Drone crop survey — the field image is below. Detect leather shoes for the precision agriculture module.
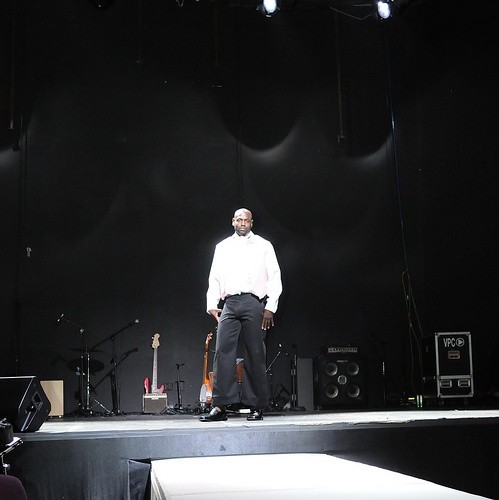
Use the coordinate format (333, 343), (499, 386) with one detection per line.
(247, 408), (263, 421)
(199, 407), (229, 422)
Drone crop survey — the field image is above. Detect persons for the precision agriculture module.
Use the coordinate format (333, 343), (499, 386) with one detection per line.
(200, 207), (282, 422)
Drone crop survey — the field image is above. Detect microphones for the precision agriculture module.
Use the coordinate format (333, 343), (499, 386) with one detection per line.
(57, 314), (64, 322)
(279, 343), (288, 355)
(128, 319), (139, 326)
(80, 328), (85, 337)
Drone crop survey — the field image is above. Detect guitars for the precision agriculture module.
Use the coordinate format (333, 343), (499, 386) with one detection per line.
(143, 332), (165, 393)
(200, 332), (212, 406)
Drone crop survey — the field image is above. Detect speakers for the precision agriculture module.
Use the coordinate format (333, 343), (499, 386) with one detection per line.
(143, 393), (167, 414)
(0, 376), (51, 432)
(317, 353), (368, 410)
